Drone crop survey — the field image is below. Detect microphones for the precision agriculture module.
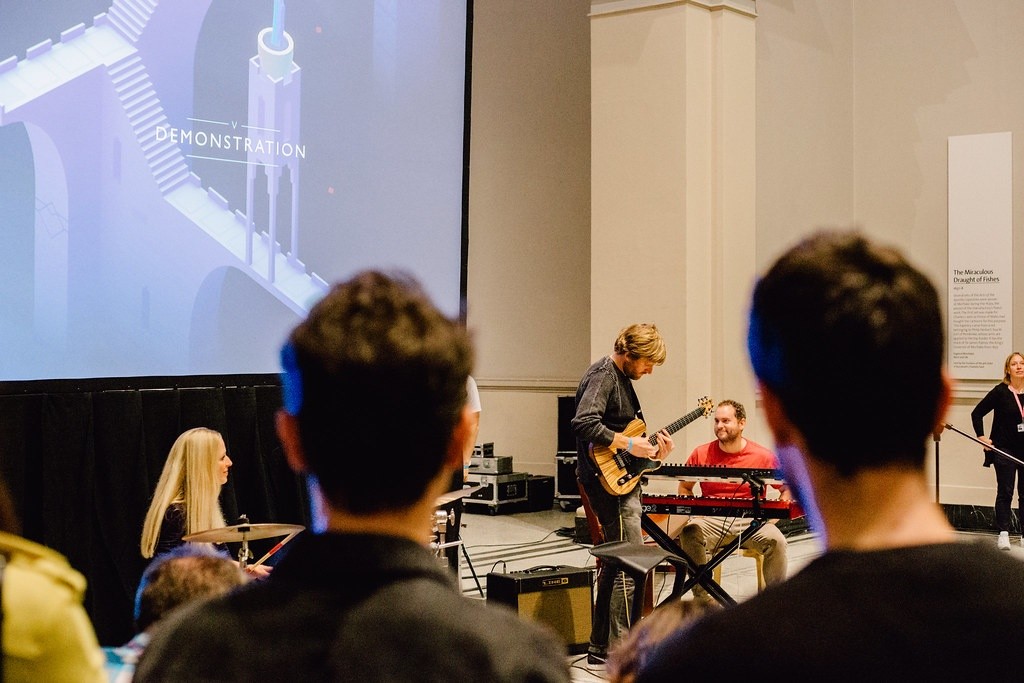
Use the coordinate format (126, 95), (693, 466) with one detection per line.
(742, 472), (761, 488)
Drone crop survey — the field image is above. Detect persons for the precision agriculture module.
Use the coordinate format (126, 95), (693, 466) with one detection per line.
(606, 599), (719, 683)
(571, 324), (676, 671)
(435, 374), (482, 591)
(0, 482), (107, 683)
(131, 271), (573, 683)
(970, 352), (1024, 550)
(138, 427), (274, 581)
(677, 400), (792, 607)
(101, 544), (241, 683)
(633, 231), (1024, 683)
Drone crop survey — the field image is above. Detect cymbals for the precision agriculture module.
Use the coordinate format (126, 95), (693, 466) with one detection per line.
(181, 514), (306, 544)
(433, 485), (487, 507)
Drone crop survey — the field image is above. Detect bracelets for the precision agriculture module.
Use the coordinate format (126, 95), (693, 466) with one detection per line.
(626, 438), (633, 452)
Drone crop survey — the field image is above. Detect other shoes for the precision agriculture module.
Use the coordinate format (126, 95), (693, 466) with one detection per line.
(588, 652), (609, 664)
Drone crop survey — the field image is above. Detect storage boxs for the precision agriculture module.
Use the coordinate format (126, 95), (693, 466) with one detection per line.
(463, 454), (580, 516)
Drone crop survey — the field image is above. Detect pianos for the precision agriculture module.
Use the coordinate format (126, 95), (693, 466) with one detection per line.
(632, 491), (808, 521)
(643, 457), (792, 488)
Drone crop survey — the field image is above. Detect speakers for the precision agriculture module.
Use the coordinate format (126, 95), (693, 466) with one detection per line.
(555, 394), (583, 454)
(486, 565), (596, 657)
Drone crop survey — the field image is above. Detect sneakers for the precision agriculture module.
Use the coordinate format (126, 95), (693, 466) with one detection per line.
(1021, 535), (1024, 547)
(997, 530), (1010, 550)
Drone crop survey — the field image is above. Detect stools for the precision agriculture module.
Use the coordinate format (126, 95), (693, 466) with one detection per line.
(711, 547), (765, 597)
(588, 540), (689, 665)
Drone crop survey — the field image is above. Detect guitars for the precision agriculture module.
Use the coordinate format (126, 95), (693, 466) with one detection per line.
(588, 395), (716, 496)
